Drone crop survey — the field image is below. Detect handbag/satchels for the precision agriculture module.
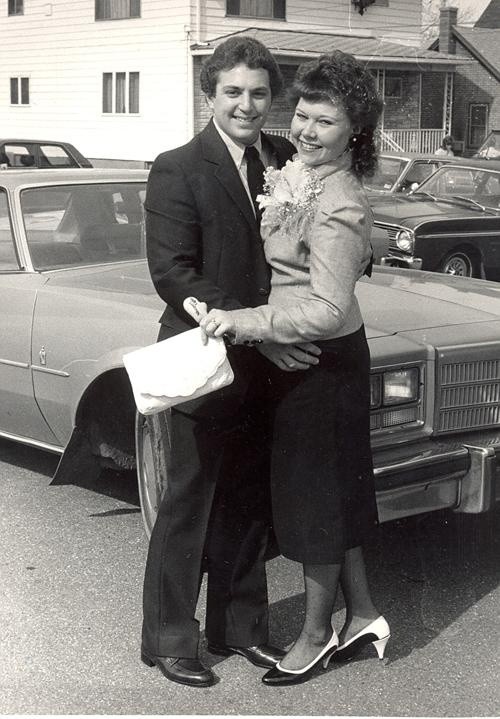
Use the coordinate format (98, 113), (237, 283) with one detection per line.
(335, 615), (391, 661)
(122, 324), (236, 417)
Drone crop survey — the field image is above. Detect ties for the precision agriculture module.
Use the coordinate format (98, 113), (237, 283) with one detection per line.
(244, 145), (266, 227)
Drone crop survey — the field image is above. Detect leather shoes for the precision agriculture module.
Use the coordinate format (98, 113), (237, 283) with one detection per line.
(140, 641), (214, 687)
(207, 638), (288, 669)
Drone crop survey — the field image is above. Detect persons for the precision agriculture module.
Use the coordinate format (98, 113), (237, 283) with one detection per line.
(138, 33), (379, 690)
(199, 46), (392, 687)
(433, 134), (457, 173)
(478, 136), (499, 158)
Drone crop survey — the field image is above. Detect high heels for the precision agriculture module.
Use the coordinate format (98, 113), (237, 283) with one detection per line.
(262, 623), (340, 686)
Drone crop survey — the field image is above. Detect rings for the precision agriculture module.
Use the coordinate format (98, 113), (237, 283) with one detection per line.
(289, 362), (296, 368)
(212, 319), (220, 327)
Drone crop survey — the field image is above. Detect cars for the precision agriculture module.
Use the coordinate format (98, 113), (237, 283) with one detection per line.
(376, 151), (482, 195)
(367, 163), (500, 278)
(0, 138), (132, 250)
(0, 167), (499, 547)
